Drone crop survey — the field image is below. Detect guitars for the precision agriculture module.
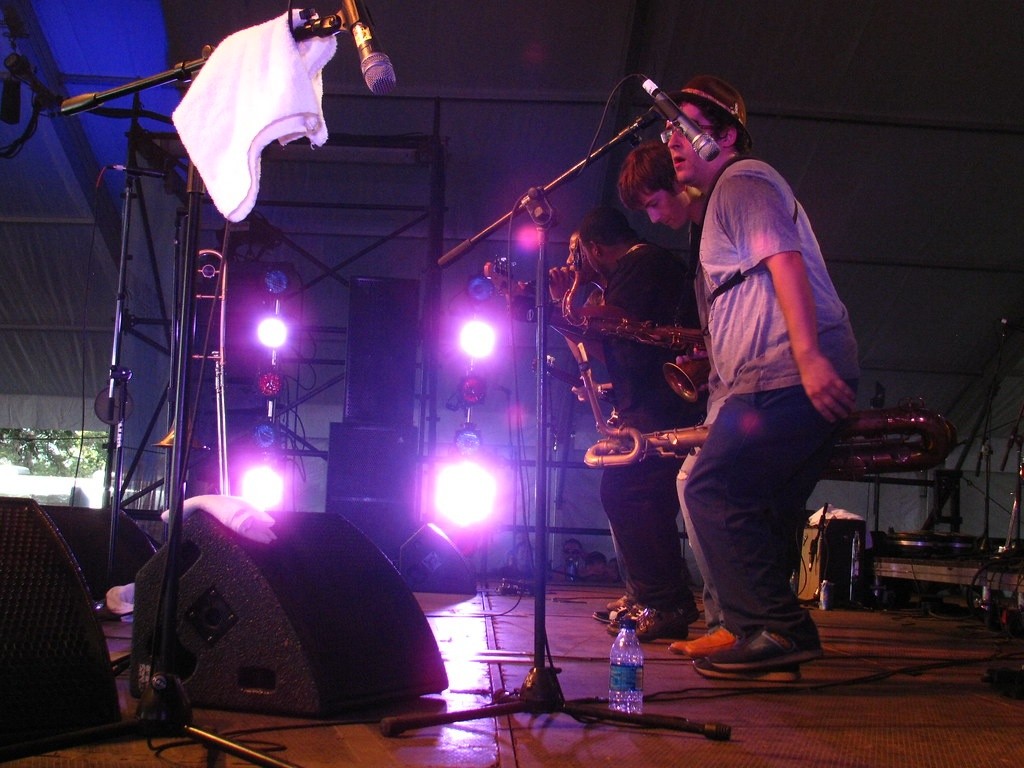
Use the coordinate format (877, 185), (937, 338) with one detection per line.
(483, 253), (608, 367)
(530, 353), (623, 427)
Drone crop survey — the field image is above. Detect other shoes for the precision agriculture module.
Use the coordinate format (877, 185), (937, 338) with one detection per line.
(607, 596), (629, 611)
(685, 627), (739, 659)
(668, 641), (693, 655)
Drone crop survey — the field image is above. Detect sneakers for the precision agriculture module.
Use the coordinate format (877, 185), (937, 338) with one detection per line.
(693, 640), (801, 682)
(607, 605), (700, 642)
(710, 628), (824, 670)
(592, 603), (646, 623)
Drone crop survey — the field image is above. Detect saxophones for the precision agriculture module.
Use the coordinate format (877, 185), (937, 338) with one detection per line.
(561, 236), (711, 403)
(575, 341), (953, 479)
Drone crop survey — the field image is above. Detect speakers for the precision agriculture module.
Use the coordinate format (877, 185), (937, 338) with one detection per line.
(327, 422), (421, 571)
(343, 277), (421, 424)
(0, 497), (478, 765)
(794, 516), (867, 605)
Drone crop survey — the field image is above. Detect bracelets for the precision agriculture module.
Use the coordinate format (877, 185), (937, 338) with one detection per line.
(598, 384), (602, 393)
(553, 296), (564, 304)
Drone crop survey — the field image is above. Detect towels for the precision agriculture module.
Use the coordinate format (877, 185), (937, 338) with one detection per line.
(808, 503), (867, 526)
(158, 493), (279, 546)
(169, 6), (340, 225)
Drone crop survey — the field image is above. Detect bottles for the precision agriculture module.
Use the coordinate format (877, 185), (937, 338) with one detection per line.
(607, 619), (644, 714)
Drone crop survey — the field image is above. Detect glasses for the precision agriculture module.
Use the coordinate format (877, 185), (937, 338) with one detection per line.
(660, 118), (726, 144)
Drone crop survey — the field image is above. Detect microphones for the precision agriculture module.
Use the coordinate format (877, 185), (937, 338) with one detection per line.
(340, 0), (396, 96)
(106, 164), (169, 179)
(809, 542), (816, 571)
(636, 75), (721, 162)
(1000, 316), (1024, 332)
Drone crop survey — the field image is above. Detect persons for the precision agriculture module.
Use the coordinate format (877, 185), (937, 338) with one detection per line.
(497, 75), (863, 682)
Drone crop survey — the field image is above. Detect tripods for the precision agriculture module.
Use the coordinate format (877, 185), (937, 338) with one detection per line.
(0, 0), (373, 768)
(381, 98), (732, 741)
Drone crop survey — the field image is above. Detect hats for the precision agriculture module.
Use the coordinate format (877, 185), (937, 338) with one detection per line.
(667, 74), (753, 155)
